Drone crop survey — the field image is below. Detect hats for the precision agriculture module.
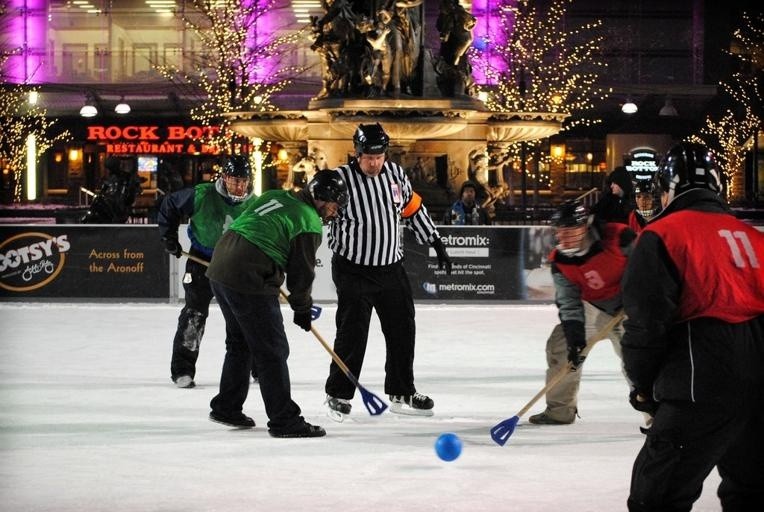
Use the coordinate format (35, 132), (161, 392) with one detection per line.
(459, 181), (477, 191)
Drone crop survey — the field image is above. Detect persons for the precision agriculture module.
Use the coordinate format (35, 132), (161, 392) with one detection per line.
(325, 124), (452, 410)
(203, 168), (350, 439)
(621, 140), (763, 511)
(528, 167), (661, 436)
(442, 181), (492, 225)
(156, 156), (261, 389)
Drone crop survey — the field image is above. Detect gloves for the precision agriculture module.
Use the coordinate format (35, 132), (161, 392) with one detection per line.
(159, 230), (183, 259)
(293, 309), (312, 332)
(568, 336), (587, 369)
(438, 243), (452, 277)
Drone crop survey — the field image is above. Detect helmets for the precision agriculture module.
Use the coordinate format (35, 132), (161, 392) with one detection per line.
(223, 154), (252, 178)
(656, 141), (726, 198)
(352, 121), (391, 155)
(634, 180), (658, 197)
(550, 199), (590, 228)
(308, 169), (349, 209)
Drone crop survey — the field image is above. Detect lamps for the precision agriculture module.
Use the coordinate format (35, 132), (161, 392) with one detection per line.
(79, 89), (98, 119)
(658, 93), (677, 118)
(621, 91), (638, 115)
(113, 90), (130, 115)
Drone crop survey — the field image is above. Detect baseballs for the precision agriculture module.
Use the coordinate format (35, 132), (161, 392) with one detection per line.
(436, 434), (461, 461)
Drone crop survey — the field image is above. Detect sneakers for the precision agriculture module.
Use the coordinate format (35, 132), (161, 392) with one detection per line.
(323, 394), (353, 414)
(529, 412), (571, 424)
(208, 409), (256, 428)
(171, 375), (196, 389)
(388, 392), (434, 409)
(267, 417), (326, 438)
